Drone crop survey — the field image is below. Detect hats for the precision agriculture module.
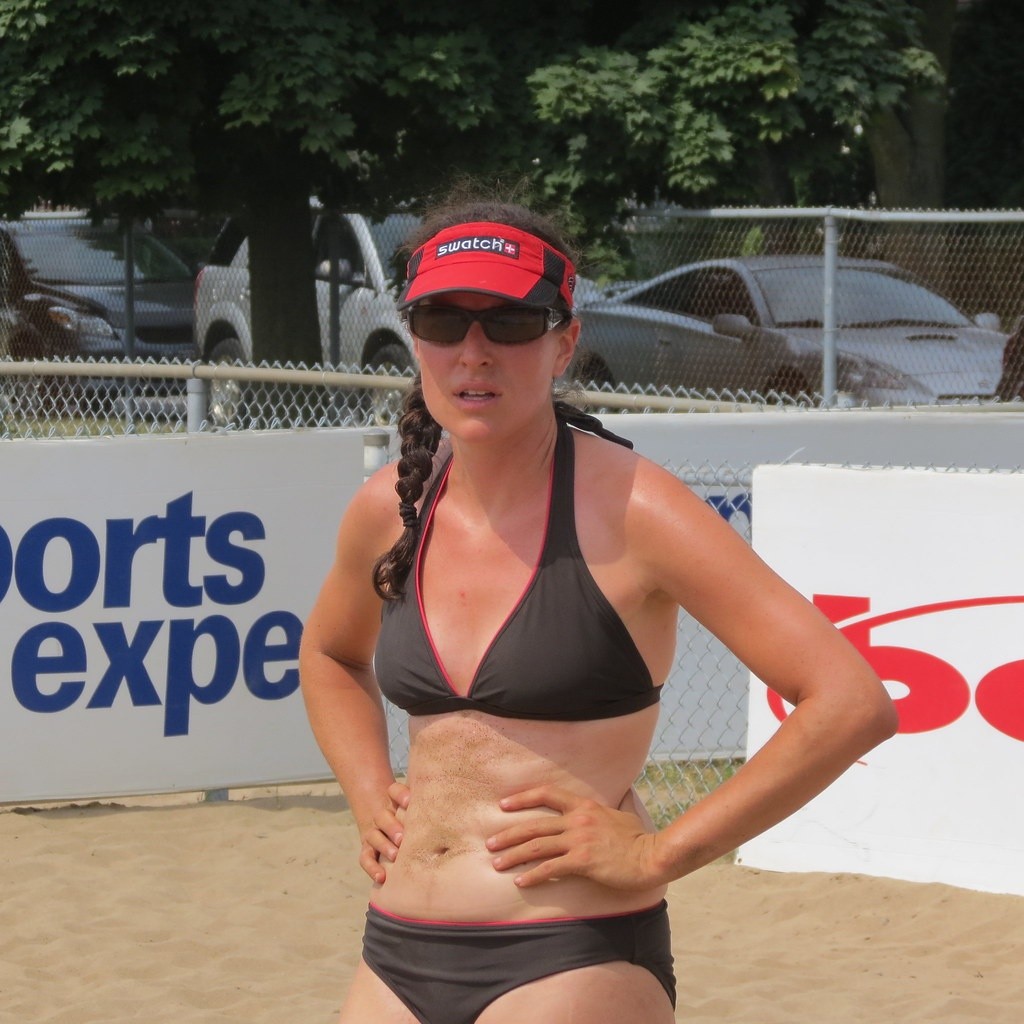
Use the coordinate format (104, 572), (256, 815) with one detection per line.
(397, 221), (578, 311)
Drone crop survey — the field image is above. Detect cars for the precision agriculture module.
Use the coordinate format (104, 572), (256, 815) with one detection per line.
(562, 253), (1012, 408)
(190, 189), (605, 430)
(0, 218), (197, 417)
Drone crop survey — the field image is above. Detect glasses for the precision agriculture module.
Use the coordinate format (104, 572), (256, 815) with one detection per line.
(401, 302), (571, 344)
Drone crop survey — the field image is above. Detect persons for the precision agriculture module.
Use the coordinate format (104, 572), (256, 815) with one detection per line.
(298, 200), (897, 1024)
